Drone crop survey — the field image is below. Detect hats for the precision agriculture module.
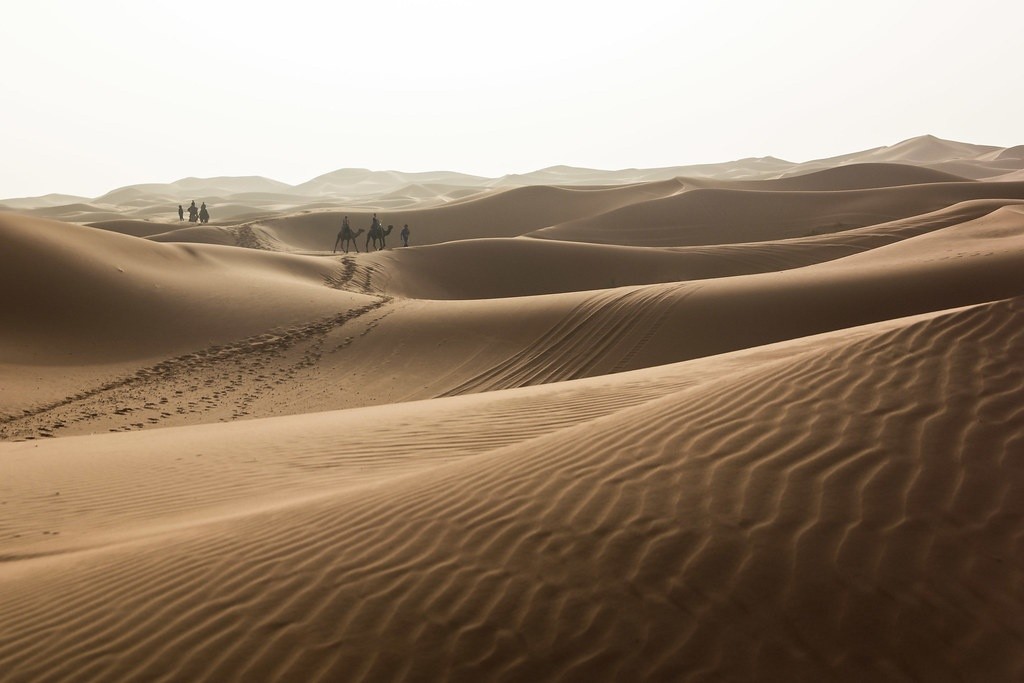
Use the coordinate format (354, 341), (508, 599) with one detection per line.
(404, 225), (408, 227)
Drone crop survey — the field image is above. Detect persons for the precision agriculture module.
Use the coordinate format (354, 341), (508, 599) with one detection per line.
(401, 225), (410, 247)
(372, 213), (380, 235)
(342, 215), (349, 239)
(179, 204), (185, 221)
(191, 200), (196, 207)
(199, 201), (206, 215)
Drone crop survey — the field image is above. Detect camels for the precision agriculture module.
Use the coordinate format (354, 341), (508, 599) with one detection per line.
(333, 228), (365, 254)
(188, 207), (209, 223)
(366, 224), (393, 252)
